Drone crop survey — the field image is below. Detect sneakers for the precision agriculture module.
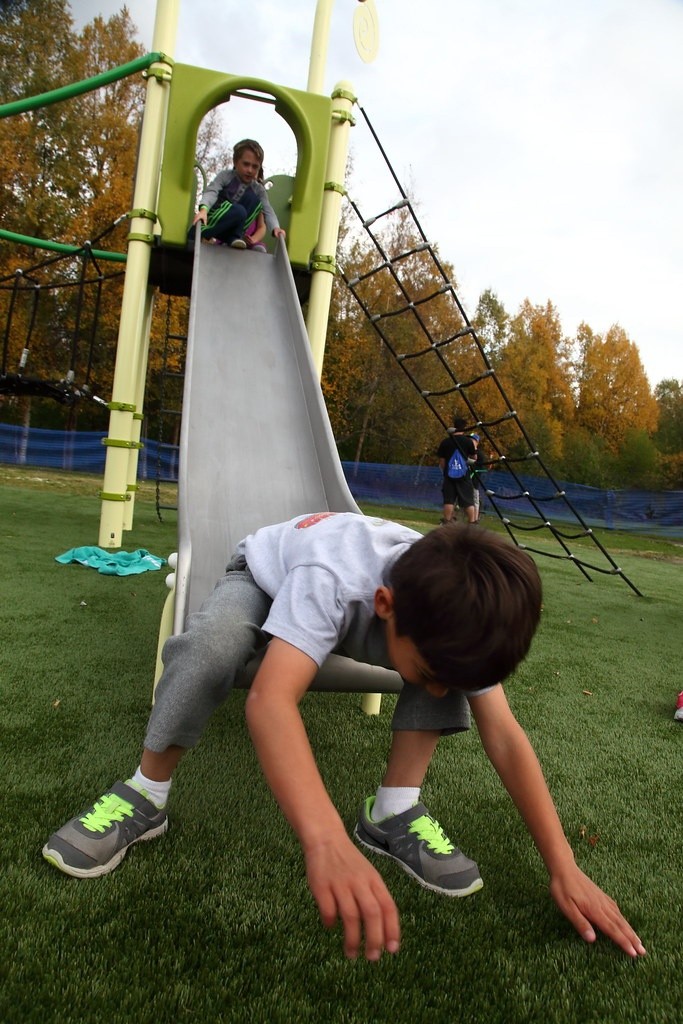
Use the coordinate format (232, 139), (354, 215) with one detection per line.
(353, 796), (483, 898)
(42, 780), (168, 879)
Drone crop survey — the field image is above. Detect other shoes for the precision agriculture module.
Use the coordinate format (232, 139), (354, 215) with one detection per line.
(253, 241), (267, 252)
(467, 519), (479, 528)
(202, 236), (221, 245)
(439, 516), (457, 526)
(231, 239), (246, 249)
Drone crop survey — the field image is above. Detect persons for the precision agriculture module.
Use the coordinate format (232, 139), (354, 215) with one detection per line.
(454, 433), (483, 522)
(437, 418), (480, 528)
(191, 139), (286, 253)
(42, 510), (647, 959)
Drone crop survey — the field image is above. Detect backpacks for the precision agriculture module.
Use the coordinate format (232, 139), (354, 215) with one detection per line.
(444, 437), (469, 479)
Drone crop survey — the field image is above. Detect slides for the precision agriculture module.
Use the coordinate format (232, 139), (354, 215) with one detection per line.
(152, 216), (406, 714)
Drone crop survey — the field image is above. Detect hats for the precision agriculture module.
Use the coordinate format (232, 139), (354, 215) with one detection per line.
(466, 433), (479, 443)
